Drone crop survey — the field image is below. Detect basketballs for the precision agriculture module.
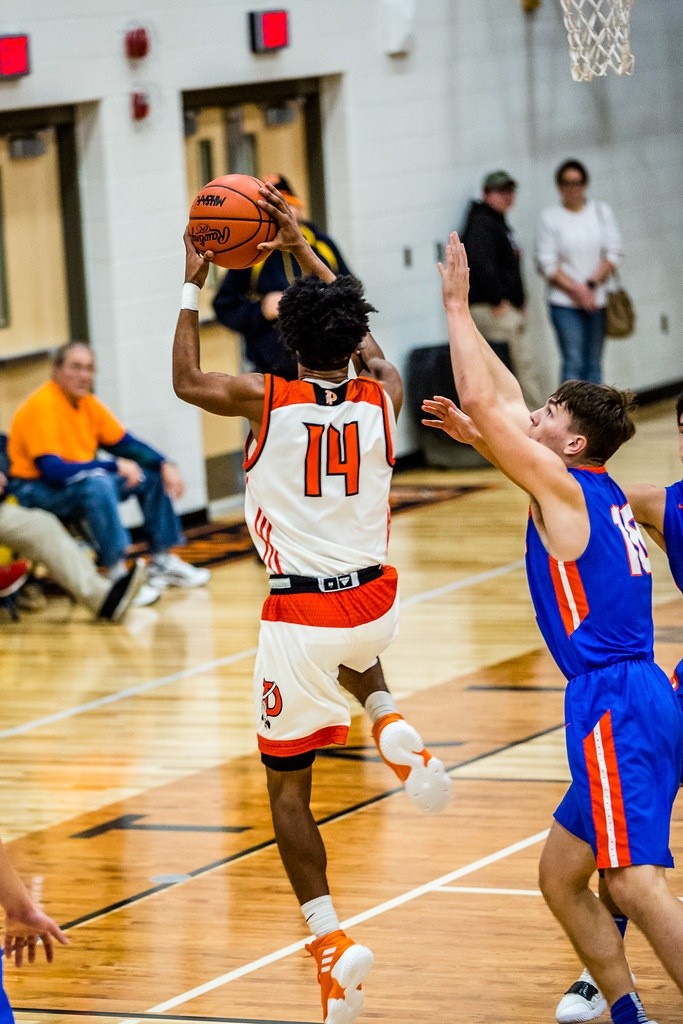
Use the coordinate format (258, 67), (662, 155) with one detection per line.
(188, 173), (278, 271)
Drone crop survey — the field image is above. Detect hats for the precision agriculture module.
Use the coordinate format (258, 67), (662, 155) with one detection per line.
(485, 172), (516, 194)
(262, 174), (304, 210)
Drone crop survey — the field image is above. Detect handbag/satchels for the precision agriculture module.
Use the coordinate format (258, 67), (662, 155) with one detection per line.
(606, 259), (634, 336)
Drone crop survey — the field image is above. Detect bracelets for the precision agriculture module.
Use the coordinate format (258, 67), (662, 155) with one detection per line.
(180, 282), (200, 312)
(585, 277), (598, 292)
(182, 280), (202, 288)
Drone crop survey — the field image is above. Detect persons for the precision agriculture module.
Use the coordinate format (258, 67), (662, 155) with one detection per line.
(420, 379), (683, 1021)
(0, 839), (70, 1023)
(1, 341), (212, 622)
(532, 158), (625, 386)
(171, 181), (451, 1024)
(458, 171), (527, 376)
(211, 175), (371, 383)
(420, 232), (683, 1024)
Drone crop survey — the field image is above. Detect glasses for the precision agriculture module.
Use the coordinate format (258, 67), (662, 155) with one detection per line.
(558, 176), (586, 189)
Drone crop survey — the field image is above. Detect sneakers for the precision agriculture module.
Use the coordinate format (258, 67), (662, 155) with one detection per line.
(147, 553), (211, 589)
(0, 562), (28, 597)
(98, 557), (145, 624)
(556, 957), (636, 1024)
(107, 570), (160, 607)
(305, 928), (374, 1024)
(370, 712), (453, 814)
